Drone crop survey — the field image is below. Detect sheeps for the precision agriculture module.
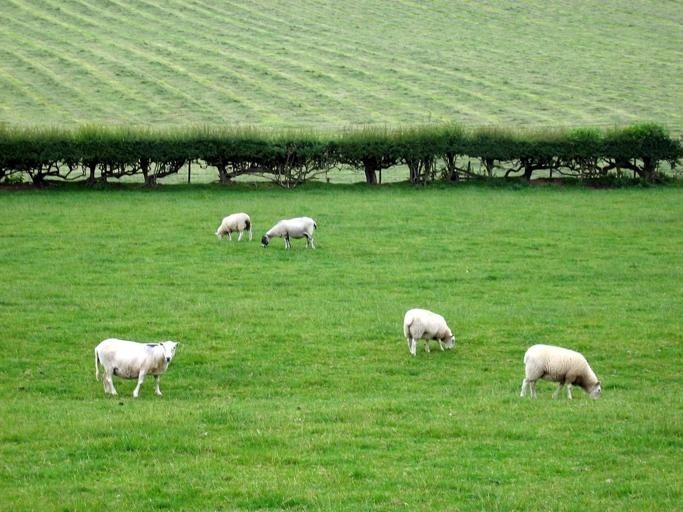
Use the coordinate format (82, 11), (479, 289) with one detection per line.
(261, 216), (317, 250)
(402, 308), (456, 358)
(214, 212), (252, 243)
(519, 344), (600, 401)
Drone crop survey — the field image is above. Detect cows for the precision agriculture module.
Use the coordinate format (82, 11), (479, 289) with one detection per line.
(94, 338), (179, 400)
